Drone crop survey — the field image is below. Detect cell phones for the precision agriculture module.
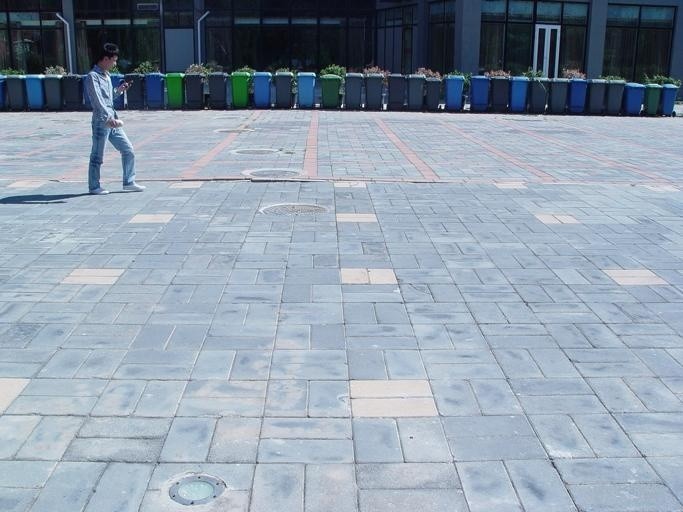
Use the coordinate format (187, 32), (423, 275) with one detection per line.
(125, 80), (133, 88)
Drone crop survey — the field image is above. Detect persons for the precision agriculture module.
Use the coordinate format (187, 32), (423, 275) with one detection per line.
(25, 46), (42, 73)
(83, 42), (146, 195)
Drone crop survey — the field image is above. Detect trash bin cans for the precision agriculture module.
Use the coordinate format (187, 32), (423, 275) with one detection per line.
(0, 71), (680, 117)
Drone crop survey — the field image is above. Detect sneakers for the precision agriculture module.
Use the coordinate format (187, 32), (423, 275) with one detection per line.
(88, 187), (110, 195)
(121, 183), (146, 192)
